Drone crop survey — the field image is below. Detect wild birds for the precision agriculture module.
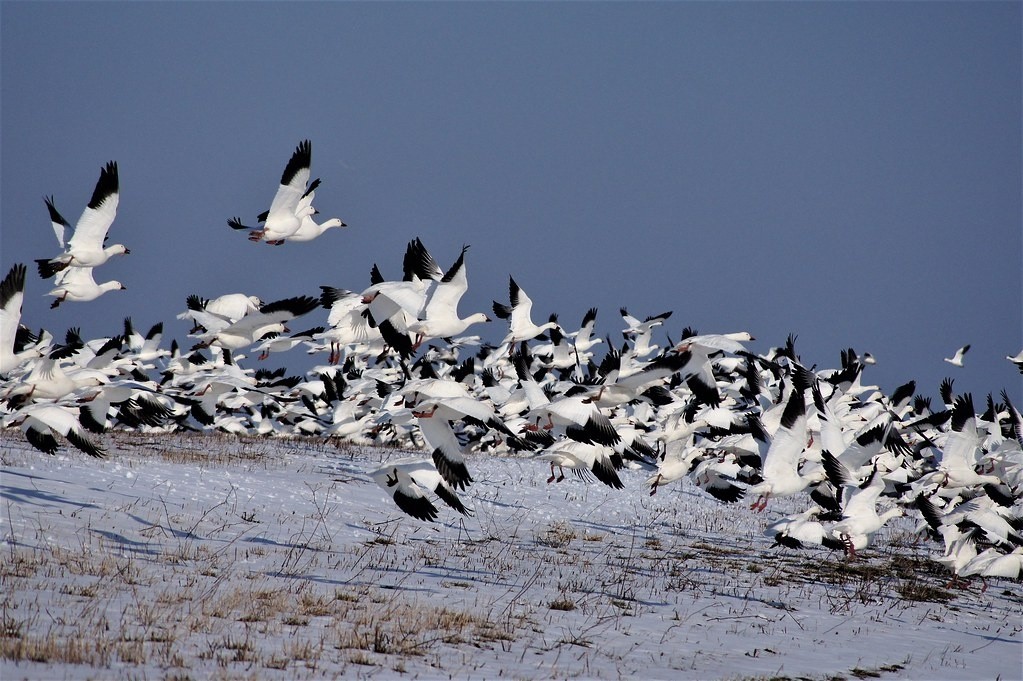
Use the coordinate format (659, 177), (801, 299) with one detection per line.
(33, 159), (131, 280)
(226, 138), (320, 243)
(256, 177), (348, 246)
(0, 235), (1022, 592)
